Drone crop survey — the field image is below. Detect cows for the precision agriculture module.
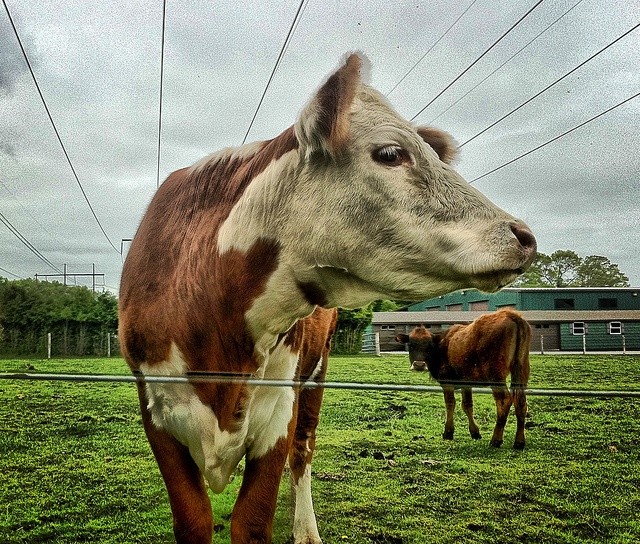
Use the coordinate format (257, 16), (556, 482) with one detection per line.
(396, 306), (533, 449)
(119, 52), (537, 544)
